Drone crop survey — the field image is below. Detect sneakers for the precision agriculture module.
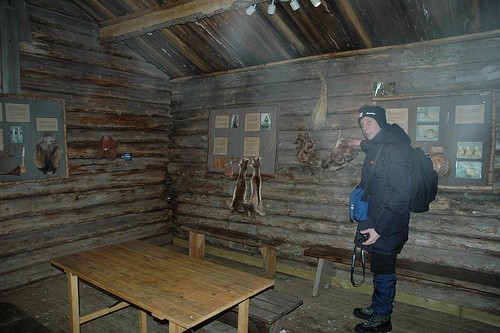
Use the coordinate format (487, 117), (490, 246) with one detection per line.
(354, 311), (392, 333)
(353, 306), (378, 319)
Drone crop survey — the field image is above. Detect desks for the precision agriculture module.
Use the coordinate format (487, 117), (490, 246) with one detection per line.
(51, 239), (276, 333)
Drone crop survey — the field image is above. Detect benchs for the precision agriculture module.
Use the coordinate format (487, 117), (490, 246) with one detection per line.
(303, 245), (500, 299)
(181, 222), (284, 285)
(0, 302), (53, 333)
(231, 288), (303, 333)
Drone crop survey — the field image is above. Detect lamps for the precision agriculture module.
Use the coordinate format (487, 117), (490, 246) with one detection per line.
(267, 0), (276, 14)
(245, 4), (257, 16)
(289, 0), (300, 11)
(311, 0), (321, 7)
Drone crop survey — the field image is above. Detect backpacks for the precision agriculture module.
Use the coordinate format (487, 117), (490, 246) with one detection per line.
(408, 145), (438, 214)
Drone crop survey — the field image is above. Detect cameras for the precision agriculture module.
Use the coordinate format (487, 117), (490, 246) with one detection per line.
(356, 233), (369, 243)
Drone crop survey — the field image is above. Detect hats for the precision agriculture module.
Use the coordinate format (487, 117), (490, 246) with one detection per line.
(357, 104), (386, 130)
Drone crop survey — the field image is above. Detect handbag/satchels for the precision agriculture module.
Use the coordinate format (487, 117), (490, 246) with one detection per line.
(349, 186), (368, 223)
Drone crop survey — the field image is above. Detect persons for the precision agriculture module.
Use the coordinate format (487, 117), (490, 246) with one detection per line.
(355, 105), (411, 333)
